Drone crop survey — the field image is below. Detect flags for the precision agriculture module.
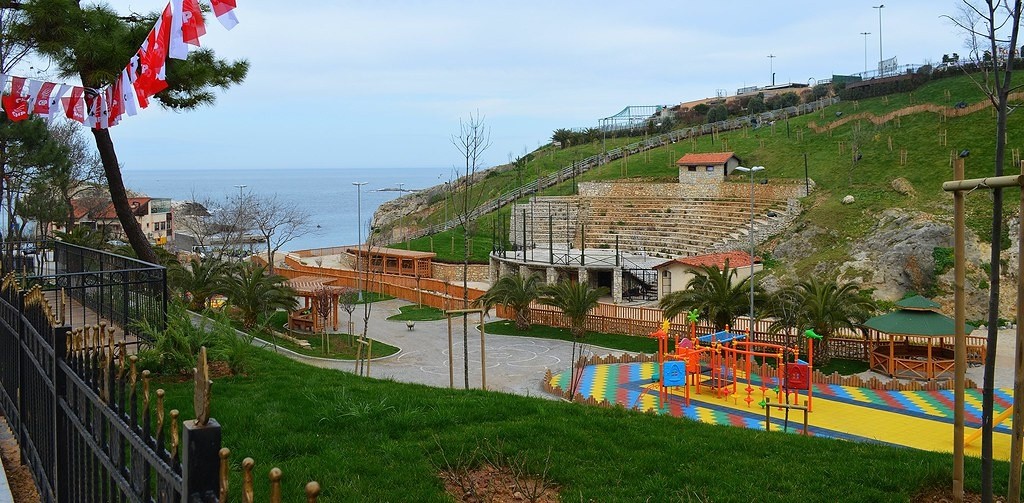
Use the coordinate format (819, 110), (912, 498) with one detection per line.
(104, 0), (240, 127)
(0, 73), (109, 130)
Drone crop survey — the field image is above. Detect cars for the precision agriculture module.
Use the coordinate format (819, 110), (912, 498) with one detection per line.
(22, 243), (41, 255)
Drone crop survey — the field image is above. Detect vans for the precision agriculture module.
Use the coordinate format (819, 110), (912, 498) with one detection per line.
(191, 244), (215, 261)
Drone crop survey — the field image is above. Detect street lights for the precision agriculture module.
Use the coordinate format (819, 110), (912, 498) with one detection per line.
(395, 182), (404, 238)
(444, 181), (449, 230)
(767, 54), (775, 86)
(860, 31), (872, 78)
(873, 4), (887, 78)
(352, 181), (370, 302)
(733, 164), (766, 362)
(233, 184), (247, 262)
